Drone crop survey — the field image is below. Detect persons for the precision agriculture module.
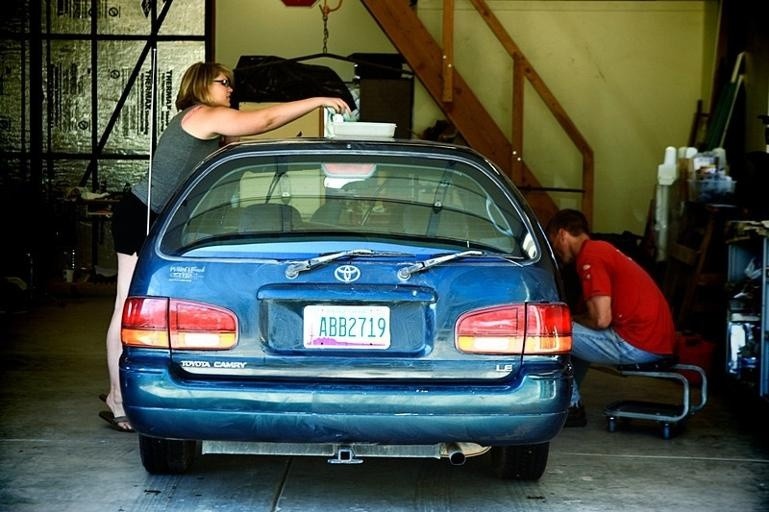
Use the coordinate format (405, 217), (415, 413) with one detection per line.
(102, 62), (351, 430)
(545, 203), (676, 426)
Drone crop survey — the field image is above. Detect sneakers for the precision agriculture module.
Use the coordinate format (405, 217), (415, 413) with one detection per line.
(562, 403), (587, 429)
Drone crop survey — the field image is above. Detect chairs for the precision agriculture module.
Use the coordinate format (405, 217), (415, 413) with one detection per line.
(240, 202), (302, 231)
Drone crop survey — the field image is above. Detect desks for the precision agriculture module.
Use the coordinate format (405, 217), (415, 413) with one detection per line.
(69, 197), (118, 296)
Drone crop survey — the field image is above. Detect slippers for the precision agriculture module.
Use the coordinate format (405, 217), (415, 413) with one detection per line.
(98, 392), (135, 434)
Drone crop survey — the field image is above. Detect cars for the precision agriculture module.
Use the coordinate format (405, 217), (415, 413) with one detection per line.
(117, 133), (576, 485)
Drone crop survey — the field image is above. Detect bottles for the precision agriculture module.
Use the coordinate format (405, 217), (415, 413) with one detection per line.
(660, 145), (698, 184)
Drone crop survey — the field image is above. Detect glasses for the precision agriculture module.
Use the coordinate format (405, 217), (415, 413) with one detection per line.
(210, 79), (231, 87)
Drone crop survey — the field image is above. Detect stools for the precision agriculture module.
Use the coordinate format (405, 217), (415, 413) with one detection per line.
(603, 355), (711, 440)
